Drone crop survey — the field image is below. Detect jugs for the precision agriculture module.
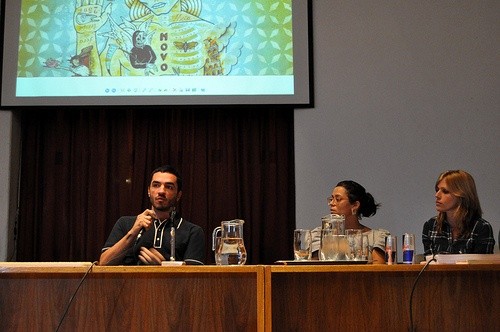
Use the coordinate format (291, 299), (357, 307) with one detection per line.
(212, 218), (247, 265)
(321, 213), (346, 261)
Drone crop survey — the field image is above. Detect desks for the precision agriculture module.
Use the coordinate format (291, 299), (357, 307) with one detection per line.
(0, 264), (500, 332)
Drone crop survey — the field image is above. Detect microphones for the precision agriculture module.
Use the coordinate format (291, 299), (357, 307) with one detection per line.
(137, 209), (155, 242)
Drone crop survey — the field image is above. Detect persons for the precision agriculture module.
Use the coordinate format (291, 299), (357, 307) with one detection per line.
(422, 170), (495, 260)
(305, 180), (392, 264)
(99, 165), (206, 266)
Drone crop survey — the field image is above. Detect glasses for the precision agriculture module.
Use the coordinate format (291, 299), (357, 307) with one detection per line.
(327, 196), (350, 204)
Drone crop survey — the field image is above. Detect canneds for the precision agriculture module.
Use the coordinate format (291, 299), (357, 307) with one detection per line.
(384, 232), (397, 264)
(402, 232), (416, 264)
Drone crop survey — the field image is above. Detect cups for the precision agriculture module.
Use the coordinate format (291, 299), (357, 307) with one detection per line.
(215, 237), (229, 265)
(385, 235), (397, 265)
(345, 229), (361, 261)
(402, 233), (415, 264)
(360, 234), (369, 260)
(294, 229), (312, 260)
(318, 229), (338, 260)
